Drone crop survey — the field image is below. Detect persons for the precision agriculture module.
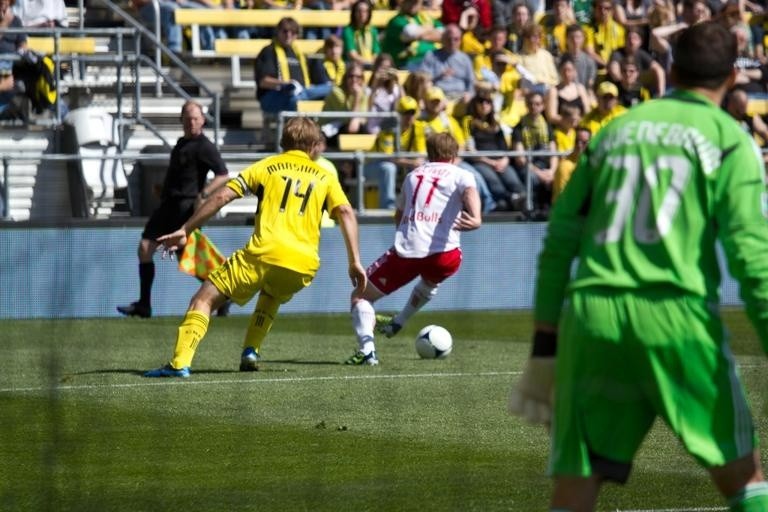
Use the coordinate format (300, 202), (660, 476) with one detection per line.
(2, 0), (768, 227)
(343, 130), (485, 368)
(504, 18), (767, 512)
(143, 114), (369, 379)
(114, 101), (234, 318)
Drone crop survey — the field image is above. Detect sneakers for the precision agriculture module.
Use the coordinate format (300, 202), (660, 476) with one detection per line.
(117, 303), (151, 319)
(240, 347), (259, 372)
(344, 351), (378, 367)
(375, 314), (401, 338)
(143, 362), (189, 379)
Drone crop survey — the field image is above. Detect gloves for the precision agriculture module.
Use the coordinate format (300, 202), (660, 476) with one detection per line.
(507, 333), (555, 426)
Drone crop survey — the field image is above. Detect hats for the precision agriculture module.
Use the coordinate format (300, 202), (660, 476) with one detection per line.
(425, 87), (443, 102)
(397, 96), (417, 113)
(598, 82), (618, 97)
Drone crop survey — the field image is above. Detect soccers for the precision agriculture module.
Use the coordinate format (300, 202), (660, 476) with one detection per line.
(416, 324), (453, 359)
